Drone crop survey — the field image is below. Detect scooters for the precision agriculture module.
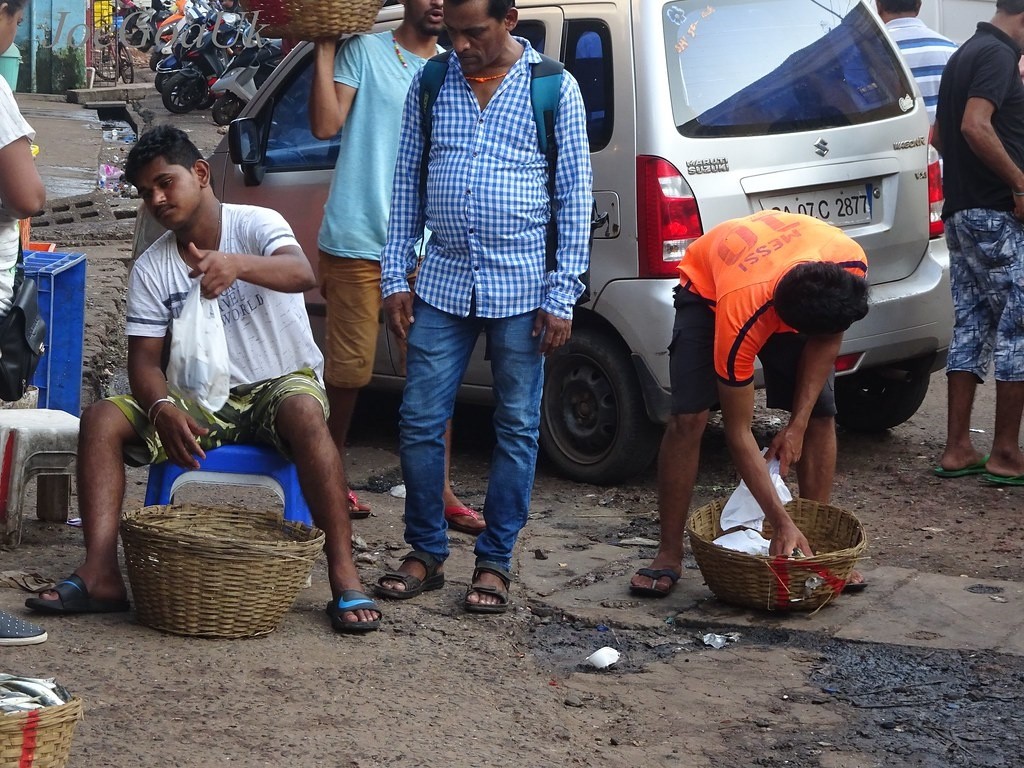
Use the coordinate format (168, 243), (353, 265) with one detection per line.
(120, 0), (284, 128)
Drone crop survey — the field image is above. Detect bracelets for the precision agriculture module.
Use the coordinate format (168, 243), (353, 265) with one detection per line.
(154, 402), (169, 425)
(147, 398), (176, 422)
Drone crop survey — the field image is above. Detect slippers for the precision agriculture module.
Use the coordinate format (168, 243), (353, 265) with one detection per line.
(933, 452), (992, 477)
(628, 568), (678, 595)
(325, 591), (383, 633)
(982, 472), (1024, 486)
(441, 505), (485, 535)
(345, 488), (370, 518)
(25, 573), (128, 614)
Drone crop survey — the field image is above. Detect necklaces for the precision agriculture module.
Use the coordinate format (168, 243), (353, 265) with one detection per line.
(464, 73), (508, 83)
(180, 200), (221, 286)
(390, 27), (439, 79)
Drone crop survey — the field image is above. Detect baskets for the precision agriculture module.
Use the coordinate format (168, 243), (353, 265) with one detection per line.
(0, 696), (82, 768)
(686, 494), (867, 610)
(118, 503), (324, 639)
(239, 0), (386, 38)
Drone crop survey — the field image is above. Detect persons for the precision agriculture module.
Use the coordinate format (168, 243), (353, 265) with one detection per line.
(928, 0), (1024, 487)
(378, 0), (594, 613)
(308, 0), (487, 536)
(630, 209), (873, 598)
(875, 0), (959, 128)
(25, 122), (383, 632)
(0, 0), (49, 647)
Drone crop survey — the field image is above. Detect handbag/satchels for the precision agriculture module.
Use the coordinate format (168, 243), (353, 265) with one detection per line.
(0, 275), (47, 401)
(166, 272), (229, 413)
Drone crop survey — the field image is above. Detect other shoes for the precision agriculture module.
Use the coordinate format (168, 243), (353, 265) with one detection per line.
(0, 609), (49, 645)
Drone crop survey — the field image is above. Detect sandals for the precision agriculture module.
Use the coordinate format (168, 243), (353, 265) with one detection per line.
(373, 551), (446, 599)
(464, 559), (515, 612)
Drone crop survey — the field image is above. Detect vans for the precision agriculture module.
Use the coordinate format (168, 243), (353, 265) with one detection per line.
(126, 0), (956, 489)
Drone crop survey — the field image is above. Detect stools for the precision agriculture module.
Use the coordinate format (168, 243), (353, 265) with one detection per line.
(0, 409), (81, 550)
(144, 445), (311, 589)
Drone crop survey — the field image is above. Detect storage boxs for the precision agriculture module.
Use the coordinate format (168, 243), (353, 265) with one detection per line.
(23, 250), (87, 414)
